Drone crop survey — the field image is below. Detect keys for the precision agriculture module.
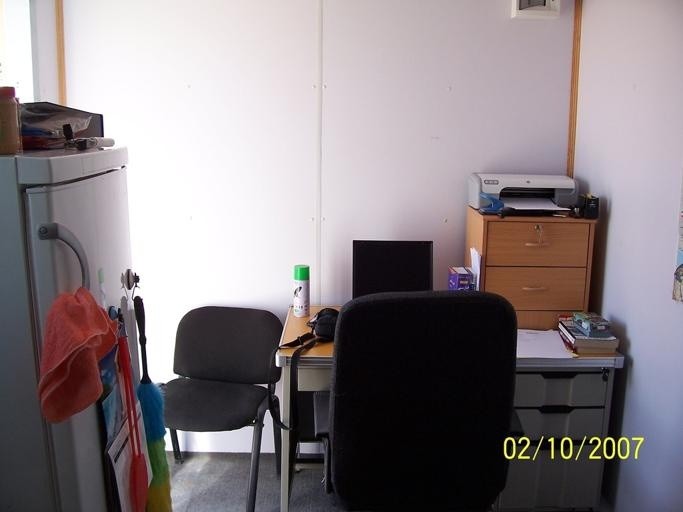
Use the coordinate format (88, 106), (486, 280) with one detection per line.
(534, 223), (543, 246)
(601, 368), (608, 387)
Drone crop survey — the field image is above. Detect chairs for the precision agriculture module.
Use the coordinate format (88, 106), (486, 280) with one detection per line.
(317, 290), (527, 512)
(154, 304), (283, 510)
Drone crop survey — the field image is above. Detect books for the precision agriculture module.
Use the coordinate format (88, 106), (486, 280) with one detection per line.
(557, 319), (619, 349)
(557, 329), (621, 350)
(464, 267), (477, 291)
(447, 266), (470, 290)
(561, 340), (621, 359)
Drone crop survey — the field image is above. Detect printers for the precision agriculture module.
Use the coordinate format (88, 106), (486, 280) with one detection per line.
(468, 172), (579, 219)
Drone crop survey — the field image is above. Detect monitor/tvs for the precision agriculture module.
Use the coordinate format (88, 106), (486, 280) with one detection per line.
(351, 239), (434, 299)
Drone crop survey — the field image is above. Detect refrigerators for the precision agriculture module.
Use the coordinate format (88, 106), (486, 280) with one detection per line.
(0, 145), (144, 512)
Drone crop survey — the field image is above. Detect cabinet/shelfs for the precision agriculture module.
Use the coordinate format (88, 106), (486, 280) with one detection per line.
(464, 202), (598, 330)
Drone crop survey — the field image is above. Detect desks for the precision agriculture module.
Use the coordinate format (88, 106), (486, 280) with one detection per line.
(278, 303), (624, 512)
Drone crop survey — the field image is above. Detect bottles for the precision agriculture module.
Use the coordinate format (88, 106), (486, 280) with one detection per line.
(293, 264), (310, 319)
(0, 86), (19, 155)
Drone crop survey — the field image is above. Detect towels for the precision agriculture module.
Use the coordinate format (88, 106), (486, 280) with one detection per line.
(36, 289), (121, 427)
